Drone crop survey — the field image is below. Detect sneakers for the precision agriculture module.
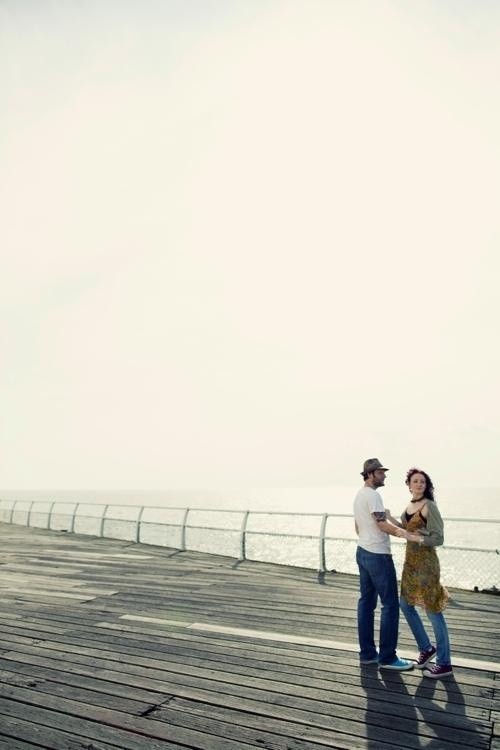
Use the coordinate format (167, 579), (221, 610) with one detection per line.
(413, 646), (437, 668)
(422, 663), (453, 678)
(360, 651), (378, 664)
(377, 657), (414, 670)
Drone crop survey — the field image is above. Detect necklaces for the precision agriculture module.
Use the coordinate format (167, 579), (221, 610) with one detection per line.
(410, 494), (425, 503)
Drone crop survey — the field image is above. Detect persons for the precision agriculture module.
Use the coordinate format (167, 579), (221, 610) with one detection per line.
(385, 468), (454, 679)
(351, 458), (422, 671)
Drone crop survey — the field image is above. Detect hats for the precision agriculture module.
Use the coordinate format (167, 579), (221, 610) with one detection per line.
(360, 458), (389, 476)
